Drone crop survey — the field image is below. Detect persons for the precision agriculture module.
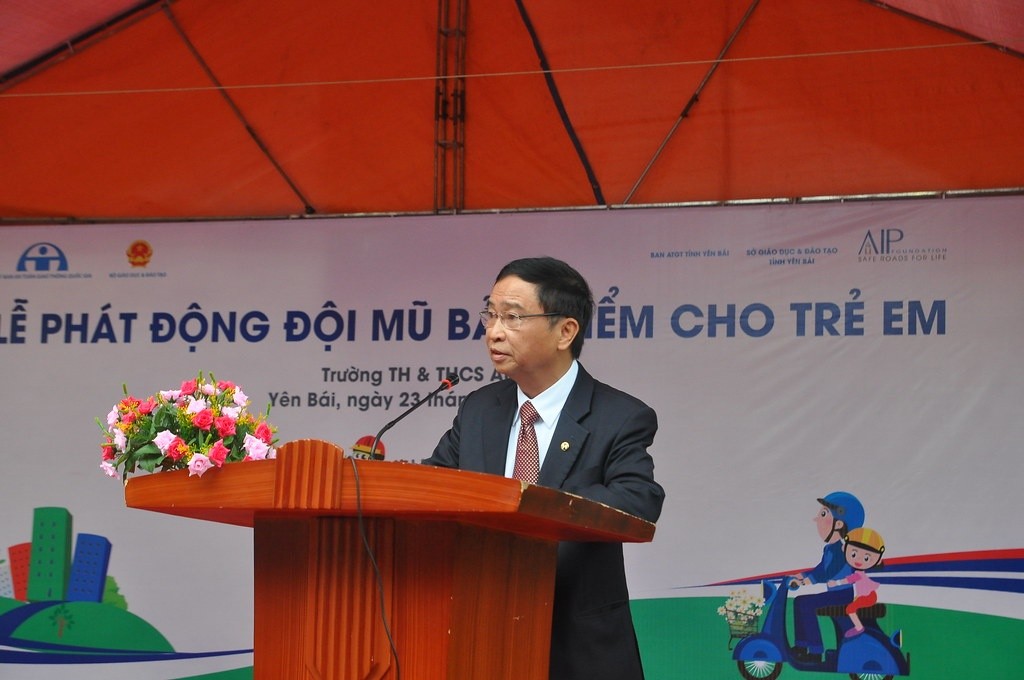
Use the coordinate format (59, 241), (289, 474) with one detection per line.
(414, 255), (667, 679)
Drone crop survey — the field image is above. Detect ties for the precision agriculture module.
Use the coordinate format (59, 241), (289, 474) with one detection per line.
(512, 401), (540, 486)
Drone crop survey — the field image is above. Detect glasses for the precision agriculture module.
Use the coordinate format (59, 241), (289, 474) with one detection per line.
(479, 310), (575, 331)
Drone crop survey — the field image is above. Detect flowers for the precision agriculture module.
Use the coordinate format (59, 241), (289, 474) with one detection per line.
(95, 369), (280, 485)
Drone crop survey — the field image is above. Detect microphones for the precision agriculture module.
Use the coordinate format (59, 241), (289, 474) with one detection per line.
(369, 373), (460, 461)
